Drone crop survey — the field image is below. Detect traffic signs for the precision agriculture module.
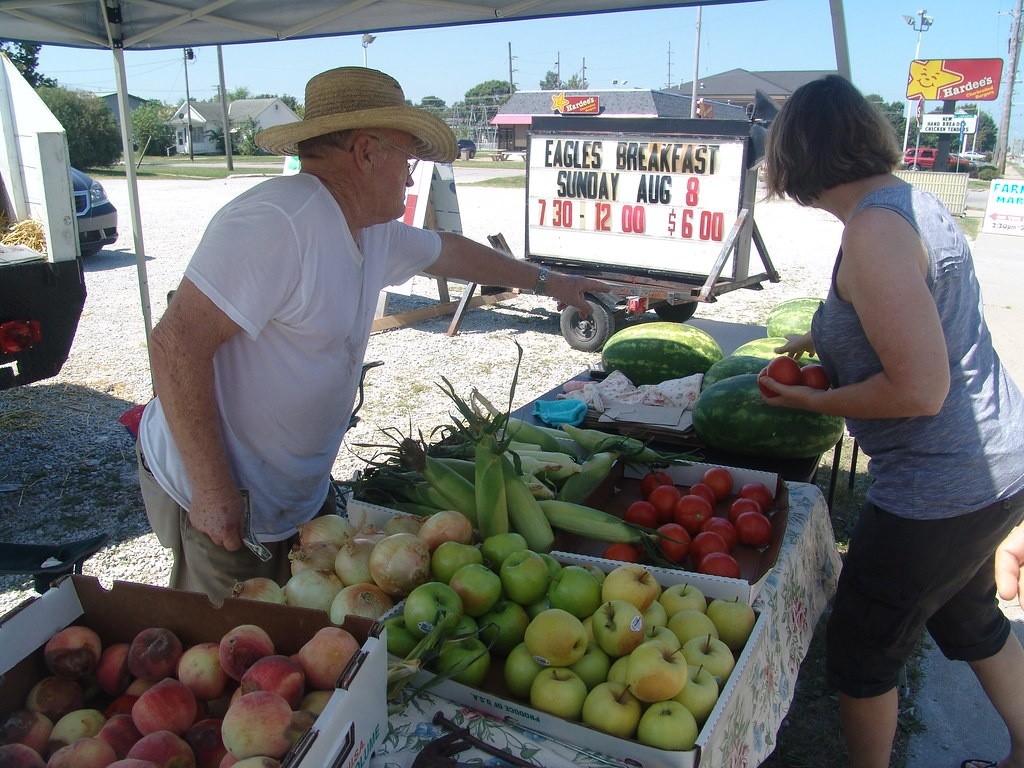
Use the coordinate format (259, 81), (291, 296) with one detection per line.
(981, 178), (1024, 237)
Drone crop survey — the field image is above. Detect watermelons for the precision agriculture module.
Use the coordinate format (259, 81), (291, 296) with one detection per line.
(602, 297), (843, 457)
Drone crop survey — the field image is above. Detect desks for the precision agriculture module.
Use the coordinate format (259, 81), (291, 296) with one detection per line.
(367, 318), (859, 768)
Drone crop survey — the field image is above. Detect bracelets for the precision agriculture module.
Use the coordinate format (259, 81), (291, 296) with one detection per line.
(533, 267), (549, 295)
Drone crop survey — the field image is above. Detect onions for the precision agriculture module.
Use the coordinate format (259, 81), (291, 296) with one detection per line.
(231, 510), (472, 625)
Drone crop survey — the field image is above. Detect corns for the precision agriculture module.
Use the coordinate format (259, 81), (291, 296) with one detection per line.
(399, 418), (659, 553)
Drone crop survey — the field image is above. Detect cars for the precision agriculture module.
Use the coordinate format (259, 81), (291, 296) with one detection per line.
(904, 148), (970, 171)
(965, 151), (986, 160)
(71, 165), (119, 256)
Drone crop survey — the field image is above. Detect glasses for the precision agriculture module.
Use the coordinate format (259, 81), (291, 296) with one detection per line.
(359, 127), (426, 177)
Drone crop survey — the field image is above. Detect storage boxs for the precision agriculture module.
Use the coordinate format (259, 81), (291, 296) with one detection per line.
(0, 463), (790, 768)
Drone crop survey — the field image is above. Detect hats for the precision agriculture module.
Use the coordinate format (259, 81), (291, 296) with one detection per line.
(253, 65), (460, 167)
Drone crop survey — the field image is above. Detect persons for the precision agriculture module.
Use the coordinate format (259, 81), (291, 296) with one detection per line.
(135, 65), (613, 594)
(760, 74), (1024, 768)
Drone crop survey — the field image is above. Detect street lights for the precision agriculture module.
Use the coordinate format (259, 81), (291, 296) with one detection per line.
(902, 9), (936, 153)
(362, 34), (378, 68)
(183, 47), (194, 160)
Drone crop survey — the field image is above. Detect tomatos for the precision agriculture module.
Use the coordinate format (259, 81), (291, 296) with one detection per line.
(758, 366), (779, 397)
(769, 356), (799, 386)
(604, 467), (771, 579)
(801, 364), (829, 390)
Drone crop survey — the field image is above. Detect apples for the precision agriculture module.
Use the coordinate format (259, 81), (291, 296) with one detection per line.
(383, 533), (755, 750)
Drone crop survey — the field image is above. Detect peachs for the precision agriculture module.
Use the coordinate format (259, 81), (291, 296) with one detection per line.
(0, 625), (359, 768)
